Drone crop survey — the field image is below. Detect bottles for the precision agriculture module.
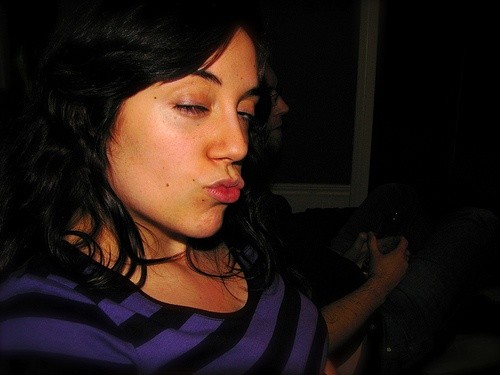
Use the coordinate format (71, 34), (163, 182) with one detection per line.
(361, 211), (400, 281)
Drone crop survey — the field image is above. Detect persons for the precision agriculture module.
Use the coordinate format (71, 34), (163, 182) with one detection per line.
(223, 65), (414, 372)
(0, 0), (336, 375)
